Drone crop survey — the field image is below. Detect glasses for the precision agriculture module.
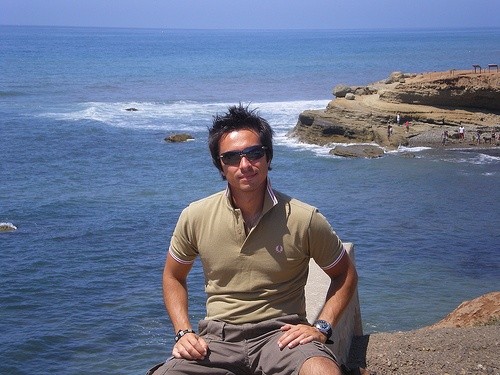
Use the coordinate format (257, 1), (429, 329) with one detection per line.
(218, 145), (267, 164)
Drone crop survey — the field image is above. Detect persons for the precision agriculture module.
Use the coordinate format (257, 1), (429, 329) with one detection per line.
(405, 120), (411, 132)
(146, 104), (359, 375)
(390, 126), (393, 133)
(396, 112), (400, 125)
(491, 127), (496, 145)
(459, 125), (466, 139)
(476, 130), (481, 145)
(387, 125), (391, 141)
(440, 131), (449, 146)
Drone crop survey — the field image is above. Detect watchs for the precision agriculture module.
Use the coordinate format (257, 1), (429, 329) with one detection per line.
(313, 319), (333, 339)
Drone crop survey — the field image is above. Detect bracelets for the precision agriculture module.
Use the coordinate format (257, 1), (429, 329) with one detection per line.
(174, 328), (195, 343)
